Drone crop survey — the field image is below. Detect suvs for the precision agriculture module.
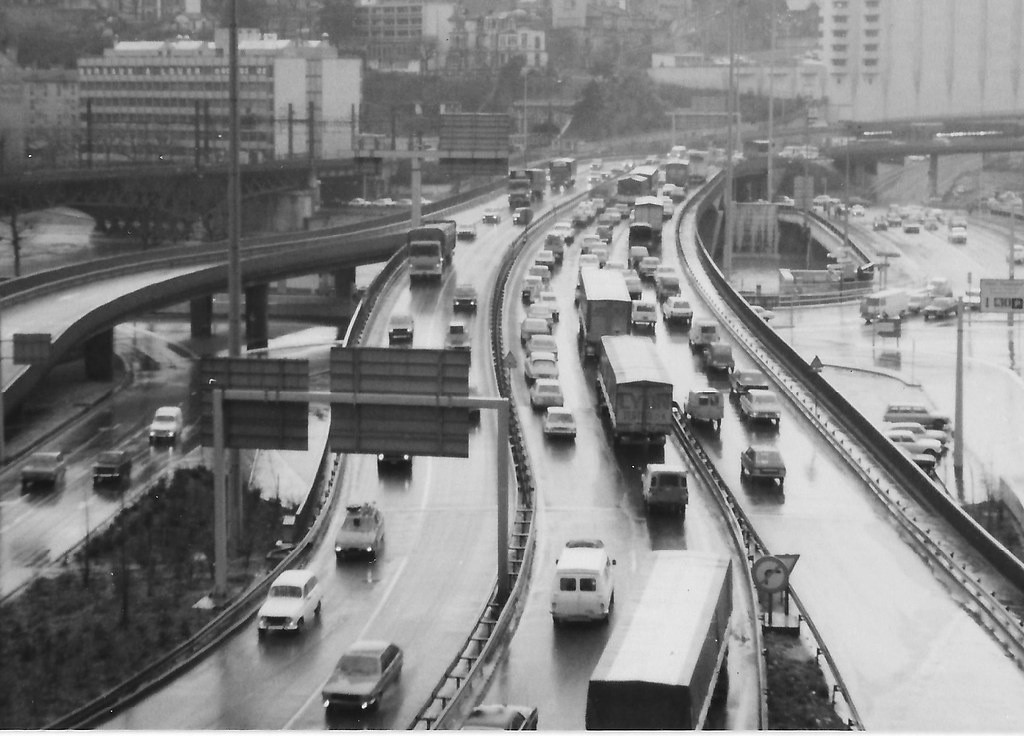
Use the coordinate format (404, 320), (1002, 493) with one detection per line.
(255, 569), (323, 635)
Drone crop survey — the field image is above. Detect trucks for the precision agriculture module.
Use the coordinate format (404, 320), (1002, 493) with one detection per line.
(595, 332), (676, 450)
(583, 549), (737, 730)
(407, 218), (459, 282)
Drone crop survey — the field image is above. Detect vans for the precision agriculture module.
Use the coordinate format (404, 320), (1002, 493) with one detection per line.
(148, 405), (184, 441)
(550, 537), (618, 625)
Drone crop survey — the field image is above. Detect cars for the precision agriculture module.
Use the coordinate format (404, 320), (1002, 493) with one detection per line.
(729, 367), (768, 393)
(455, 282), (480, 312)
(740, 446), (786, 482)
(92, 450), (131, 482)
(376, 454), (413, 468)
(483, 212), (502, 224)
(320, 640), (405, 713)
(764, 192), (1023, 472)
(740, 389), (781, 422)
(388, 313), (413, 343)
(639, 463), (691, 514)
(544, 408), (574, 439)
(504, 142), (736, 382)
(460, 702), (539, 731)
(458, 220), (478, 238)
(19, 450), (66, 487)
(530, 379), (564, 408)
(333, 503), (384, 560)
